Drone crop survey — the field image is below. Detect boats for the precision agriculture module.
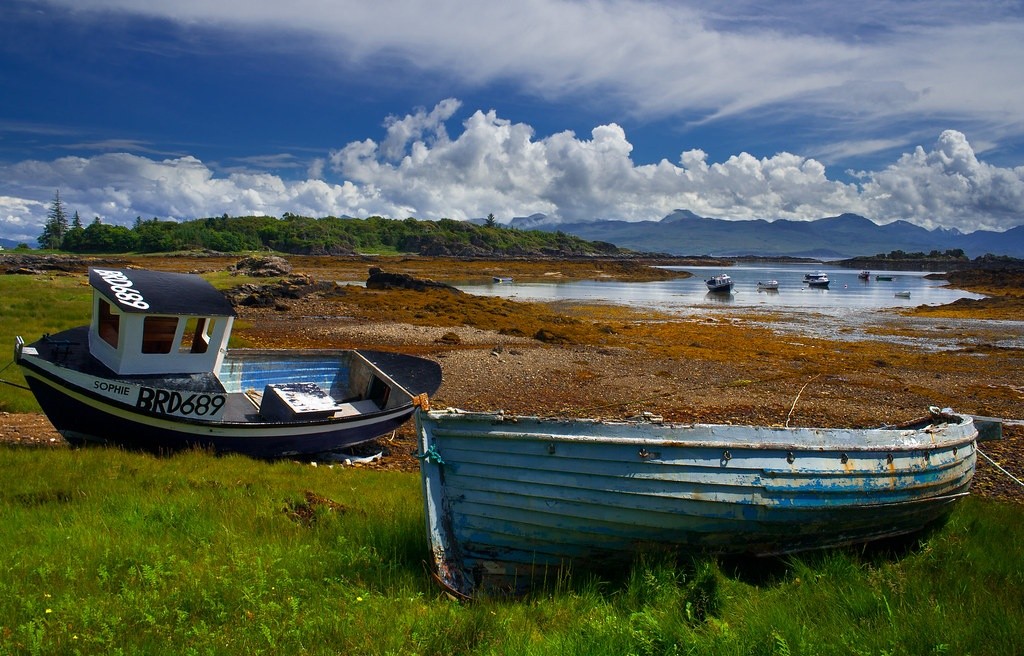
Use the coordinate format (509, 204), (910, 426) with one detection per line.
(858, 270), (870, 278)
(14, 267), (443, 460)
(876, 274), (893, 280)
(705, 272), (734, 292)
(895, 291), (910, 297)
(756, 280), (778, 289)
(803, 270), (830, 287)
(412, 393), (980, 601)
(493, 276), (513, 282)
